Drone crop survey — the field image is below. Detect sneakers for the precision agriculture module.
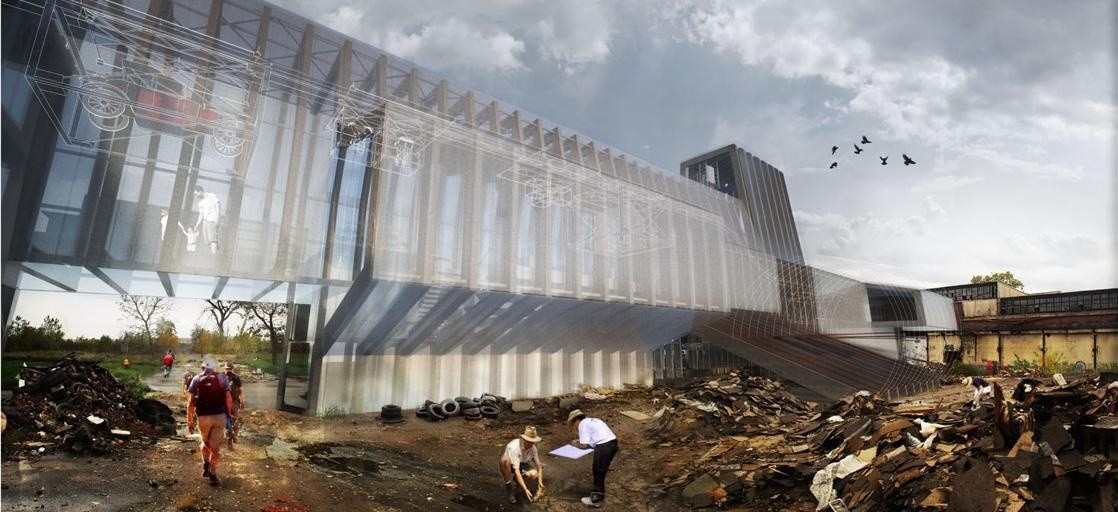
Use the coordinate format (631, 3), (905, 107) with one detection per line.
(581, 497), (601, 507)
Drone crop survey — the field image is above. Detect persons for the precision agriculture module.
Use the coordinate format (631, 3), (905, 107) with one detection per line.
(567, 408), (619, 508)
(187, 353), (233, 481)
(500, 425), (545, 504)
(163, 349), (174, 376)
(183, 365), (196, 396)
(224, 362), (244, 448)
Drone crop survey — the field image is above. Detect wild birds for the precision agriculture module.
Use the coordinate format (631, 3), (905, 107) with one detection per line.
(831, 146), (839, 155)
(831, 162), (838, 169)
(880, 156), (889, 165)
(903, 154), (916, 166)
(861, 135), (872, 144)
(854, 144), (864, 154)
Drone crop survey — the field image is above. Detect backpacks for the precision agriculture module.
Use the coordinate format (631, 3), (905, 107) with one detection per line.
(199, 375), (222, 412)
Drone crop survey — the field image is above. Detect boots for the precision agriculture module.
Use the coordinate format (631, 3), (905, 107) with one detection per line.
(203, 459), (218, 481)
(506, 481), (517, 503)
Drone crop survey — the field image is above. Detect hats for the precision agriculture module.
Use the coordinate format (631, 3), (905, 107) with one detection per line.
(201, 358), (218, 370)
(568, 409), (584, 421)
(225, 362), (233, 368)
(520, 426), (542, 442)
